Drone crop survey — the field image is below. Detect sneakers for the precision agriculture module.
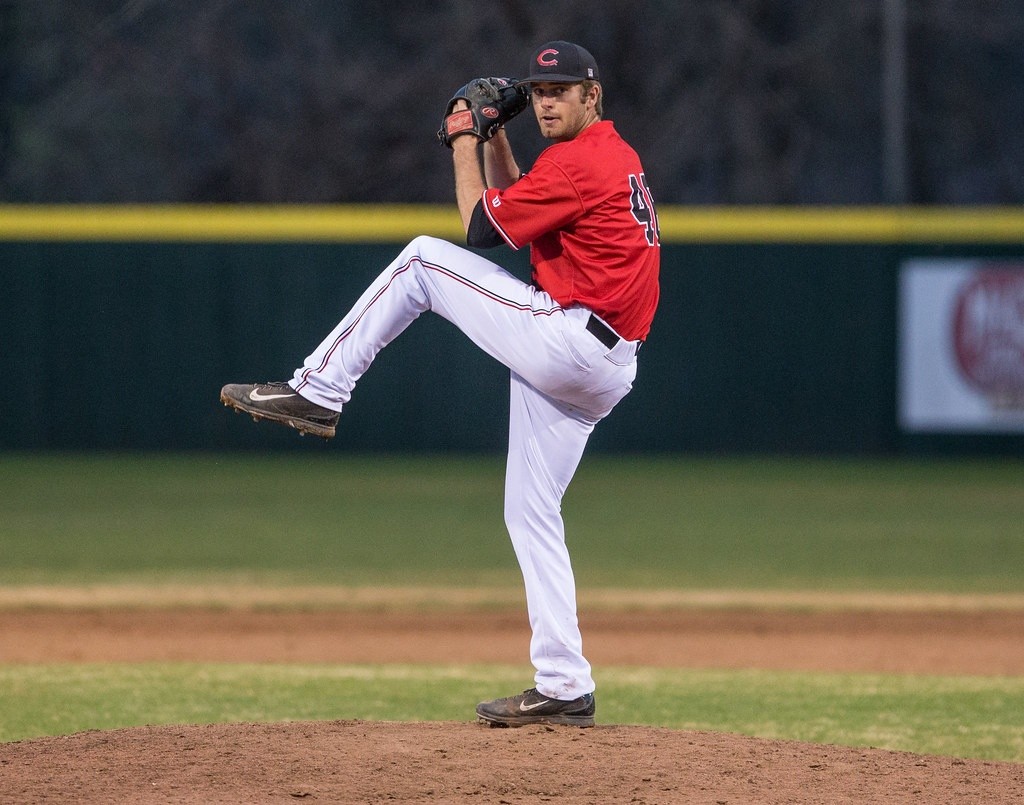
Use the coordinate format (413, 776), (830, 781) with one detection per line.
(221, 382), (340, 440)
(476, 685), (596, 726)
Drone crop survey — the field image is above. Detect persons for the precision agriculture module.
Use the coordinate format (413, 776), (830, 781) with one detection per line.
(220, 40), (661, 727)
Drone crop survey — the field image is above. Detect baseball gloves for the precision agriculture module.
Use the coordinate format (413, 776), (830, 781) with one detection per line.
(439, 76), (530, 148)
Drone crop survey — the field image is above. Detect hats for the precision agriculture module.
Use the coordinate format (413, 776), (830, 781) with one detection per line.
(515, 41), (600, 83)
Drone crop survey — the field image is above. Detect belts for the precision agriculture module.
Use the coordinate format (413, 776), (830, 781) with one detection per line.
(587, 315), (642, 357)
